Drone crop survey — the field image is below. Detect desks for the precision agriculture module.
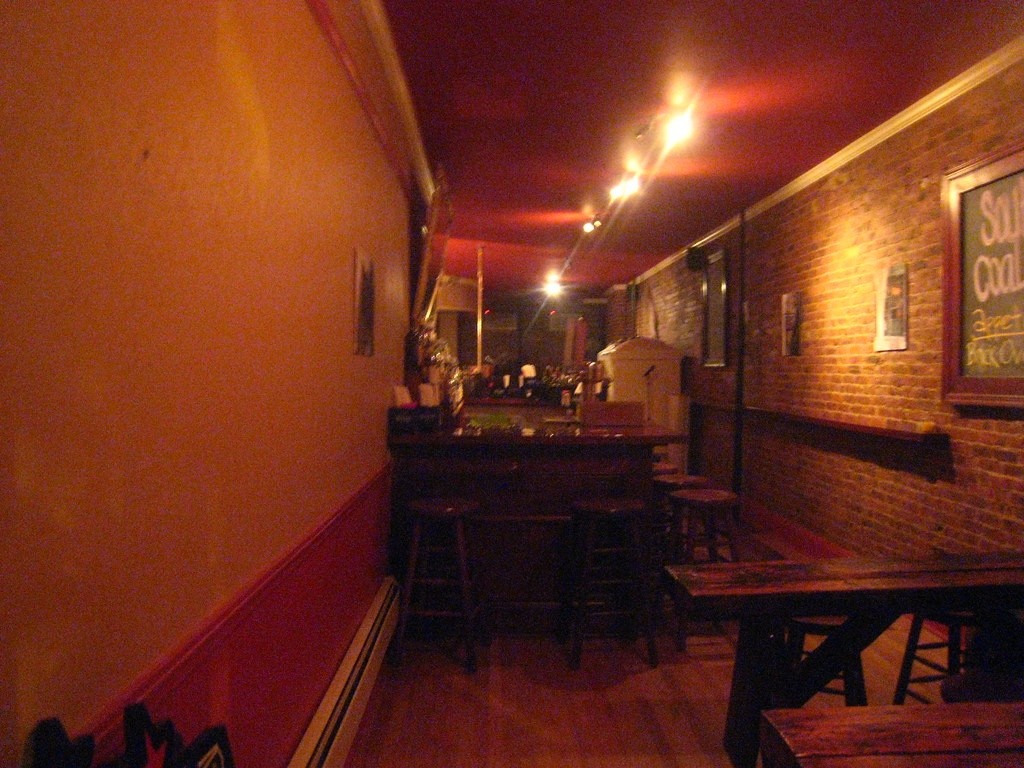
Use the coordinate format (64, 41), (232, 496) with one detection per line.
(663, 549), (1024, 767)
(758, 701), (1023, 768)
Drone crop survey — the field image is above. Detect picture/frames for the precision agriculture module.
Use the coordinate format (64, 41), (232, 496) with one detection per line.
(701, 249), (727, 368)
(940, 141), (1024, 408)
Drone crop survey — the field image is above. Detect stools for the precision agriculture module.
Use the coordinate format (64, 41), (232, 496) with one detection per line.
(784, 615), (868, 708)
(563, 497), (655, 669)
(893, 608), (980, 707)
(651, 458), (744, 653)
(394, 495), (490, 674)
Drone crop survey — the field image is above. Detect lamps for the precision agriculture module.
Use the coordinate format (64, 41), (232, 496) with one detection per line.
(581, 214), (603, 232)
